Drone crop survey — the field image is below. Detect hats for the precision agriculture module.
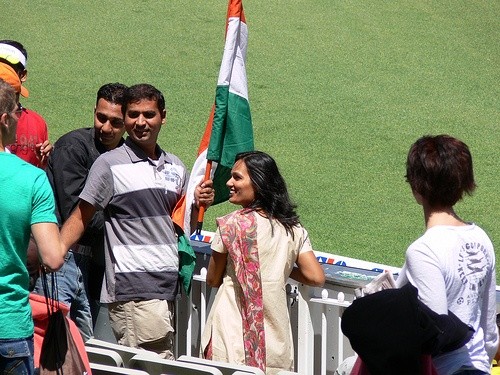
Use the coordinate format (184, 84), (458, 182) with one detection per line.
(0, 62), (29, 98)
(0, 43), (27, 69)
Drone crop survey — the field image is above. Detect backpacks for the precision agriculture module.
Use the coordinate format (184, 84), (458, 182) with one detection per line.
(39, 263), (88, 375)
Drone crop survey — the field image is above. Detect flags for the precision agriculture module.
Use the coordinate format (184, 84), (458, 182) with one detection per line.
(169, 0), (254, 299)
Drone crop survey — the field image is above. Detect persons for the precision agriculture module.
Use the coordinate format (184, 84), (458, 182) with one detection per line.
(395, 135), (500, 375)
(0, 39), (54, 290)
(62, 83), (215, 359)
(33, 82), (129, 341)
(201, 151), (327, 373)
(0, 78), (64, 375)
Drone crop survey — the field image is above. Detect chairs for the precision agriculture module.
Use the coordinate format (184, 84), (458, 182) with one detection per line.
(85, 339), (265, 375)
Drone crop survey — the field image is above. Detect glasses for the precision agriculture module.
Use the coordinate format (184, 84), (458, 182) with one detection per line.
(8, 107), (22, 118)
